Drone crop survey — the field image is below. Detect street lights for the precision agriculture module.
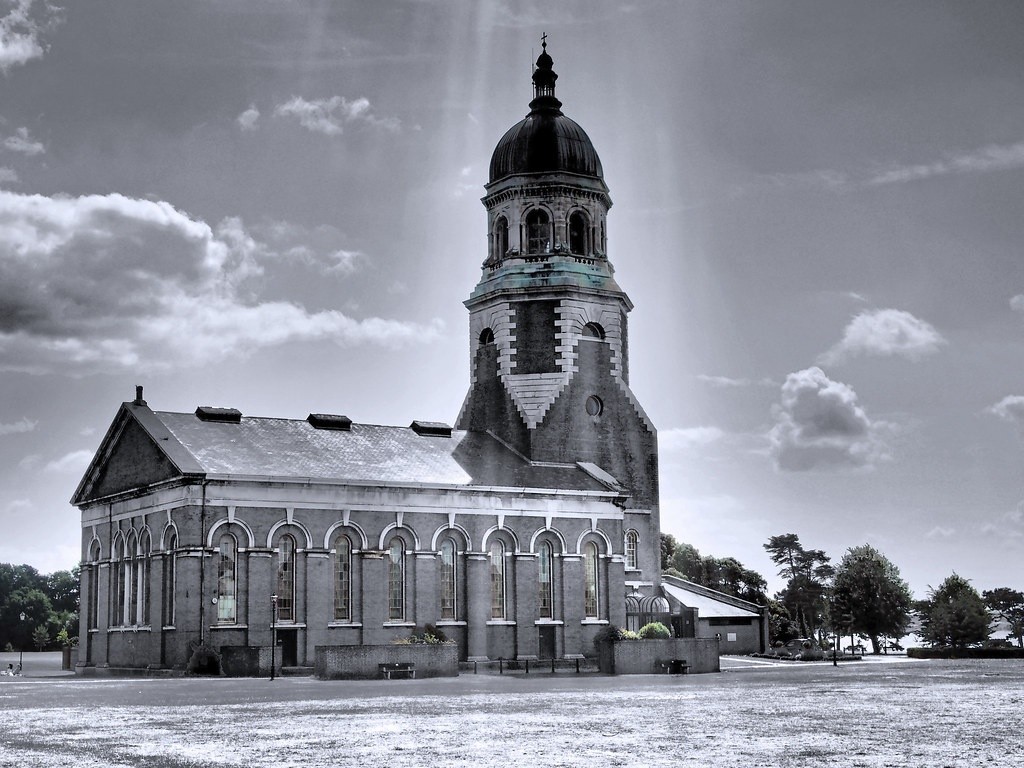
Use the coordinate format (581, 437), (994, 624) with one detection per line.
(19, 612), (25, 671)
(270, 595), (278, 680)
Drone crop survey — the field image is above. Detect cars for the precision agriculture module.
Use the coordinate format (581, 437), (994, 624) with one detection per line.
(778, 639), (823, 660)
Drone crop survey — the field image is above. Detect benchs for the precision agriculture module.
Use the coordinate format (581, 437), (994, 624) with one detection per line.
(378, 662), (417, 680)
(658, 659), (692, 675)
